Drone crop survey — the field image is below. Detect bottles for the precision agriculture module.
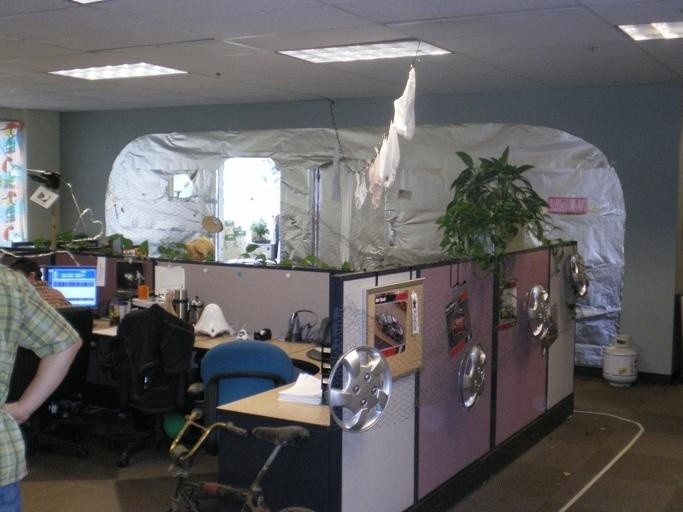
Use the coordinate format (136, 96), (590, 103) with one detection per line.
(190, 294), (203, 326)
(175, 288), (188, 323)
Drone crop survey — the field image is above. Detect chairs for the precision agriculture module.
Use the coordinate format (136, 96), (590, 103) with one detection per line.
(203, 338), (292, 465)
(11, 307), (98, 457)
(113, 306), (204, 463)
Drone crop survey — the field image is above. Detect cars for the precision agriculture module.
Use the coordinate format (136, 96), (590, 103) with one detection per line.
(374, 310), (404, 343)
(450, 310), (469, 337)
(500, 304), (516, 320)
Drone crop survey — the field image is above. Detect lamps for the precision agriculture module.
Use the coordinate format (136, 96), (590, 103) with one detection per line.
(25, 169), (90, 251)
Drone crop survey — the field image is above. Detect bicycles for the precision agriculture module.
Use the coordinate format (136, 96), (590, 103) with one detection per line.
(164, 405), (316, 512)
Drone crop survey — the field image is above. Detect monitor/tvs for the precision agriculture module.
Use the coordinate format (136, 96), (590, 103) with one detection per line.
(109, 234), (123, 255)
(12, 242), (47, 249)
(47, 266), (99, 310)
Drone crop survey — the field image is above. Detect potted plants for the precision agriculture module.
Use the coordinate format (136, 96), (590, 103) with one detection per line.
(435, 144), (568, 292)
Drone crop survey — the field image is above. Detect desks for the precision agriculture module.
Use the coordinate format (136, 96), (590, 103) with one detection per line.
(81, 315), (333, 512)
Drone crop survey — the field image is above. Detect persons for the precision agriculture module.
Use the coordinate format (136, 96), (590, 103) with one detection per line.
(0, 260), (83, 511)
(134, 269), (145, 285)
(6, 258), (70, 309)
